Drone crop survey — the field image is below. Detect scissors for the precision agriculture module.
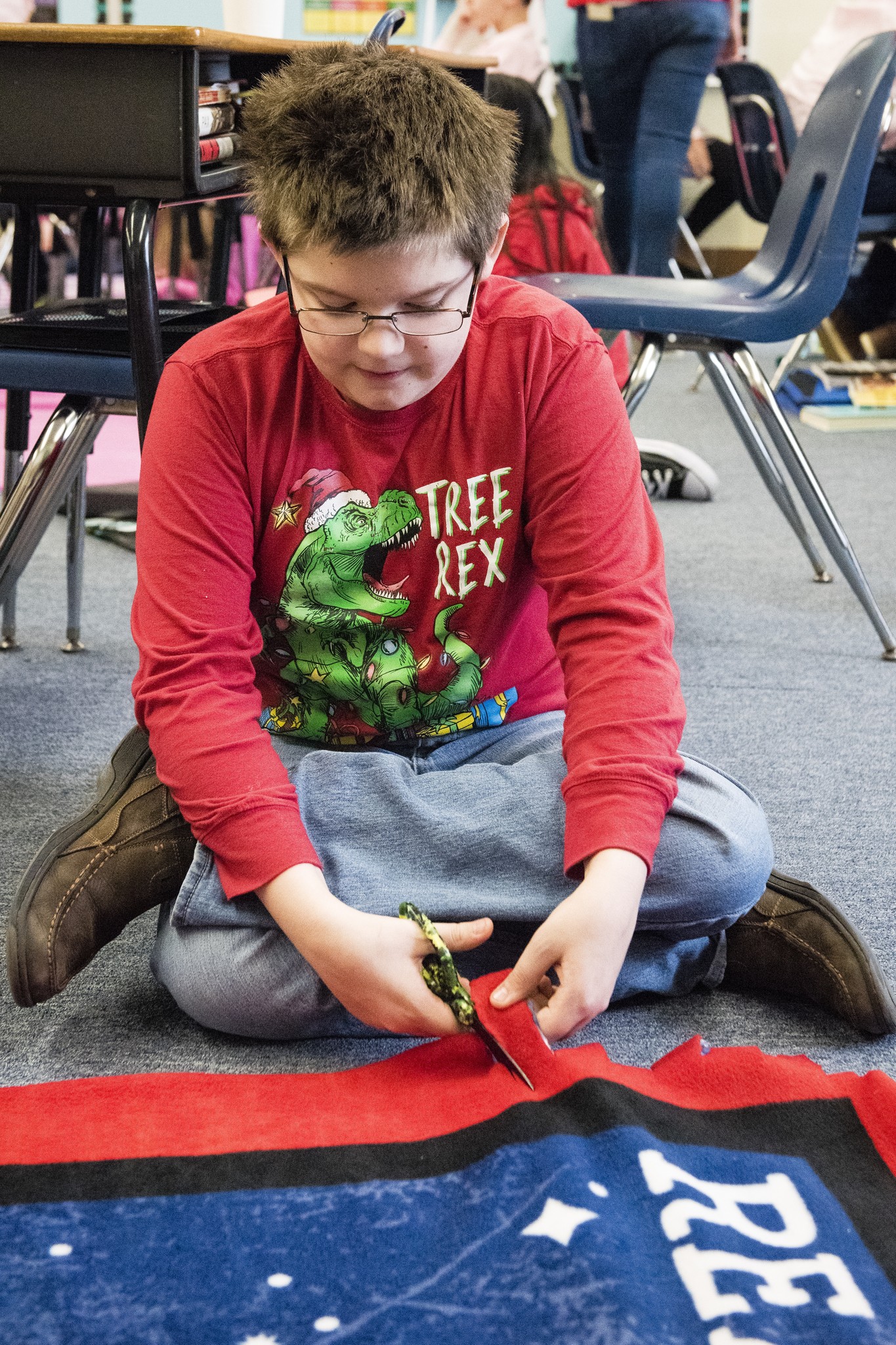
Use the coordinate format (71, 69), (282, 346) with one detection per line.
(399, 900), (538, 1093)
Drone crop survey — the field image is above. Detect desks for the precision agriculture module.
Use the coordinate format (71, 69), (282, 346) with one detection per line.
(0, 35), (501, 653)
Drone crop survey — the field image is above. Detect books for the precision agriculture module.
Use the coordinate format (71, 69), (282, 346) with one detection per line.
(199, 86), (243, 166)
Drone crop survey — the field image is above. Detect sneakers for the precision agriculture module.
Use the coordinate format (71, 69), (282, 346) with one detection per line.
(9, 724), (199, 1007)
(633, 439), (717, 501)
(721, 873), (895, 1036)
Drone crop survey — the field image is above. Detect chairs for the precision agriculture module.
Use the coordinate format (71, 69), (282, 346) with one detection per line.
(0, 12), (896, 660)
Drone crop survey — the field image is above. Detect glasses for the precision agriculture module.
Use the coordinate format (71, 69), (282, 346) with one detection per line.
(282, 248), (484, 337)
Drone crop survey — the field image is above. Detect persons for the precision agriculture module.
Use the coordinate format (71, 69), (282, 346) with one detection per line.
(484, 73), (720, 500)
(567, 0), (745, 355)
(9, 41), (895, 1052)
(438, 0), (557, 119)
(779, 0), (896, 335)
